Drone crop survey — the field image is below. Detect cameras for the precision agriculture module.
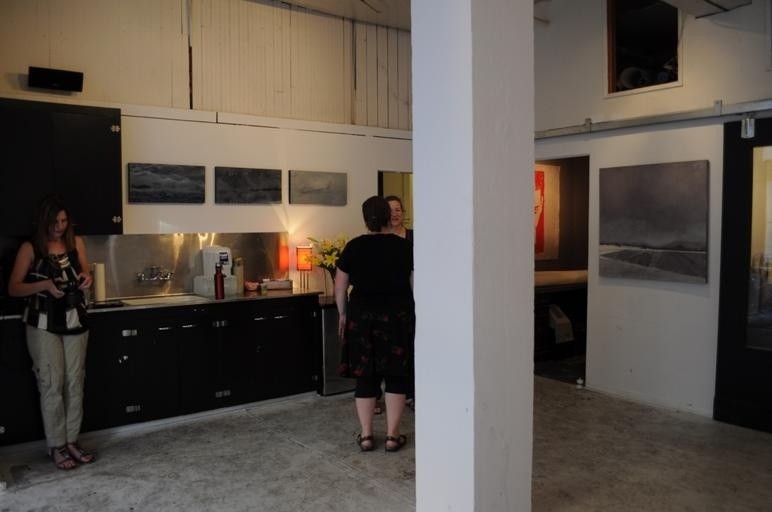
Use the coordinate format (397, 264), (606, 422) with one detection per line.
(56, 277), (85, 306)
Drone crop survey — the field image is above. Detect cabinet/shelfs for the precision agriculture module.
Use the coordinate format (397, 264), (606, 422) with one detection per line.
(2, 294), (317, 451)
(1, 97), (125, 235)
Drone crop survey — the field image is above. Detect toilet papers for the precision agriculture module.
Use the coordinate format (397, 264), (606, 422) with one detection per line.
(93, 263), (106, 302)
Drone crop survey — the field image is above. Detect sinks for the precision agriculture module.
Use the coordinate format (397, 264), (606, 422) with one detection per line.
(119, 295), (211, 306)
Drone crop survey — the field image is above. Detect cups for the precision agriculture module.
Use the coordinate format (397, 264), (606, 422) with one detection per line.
(260, 283), (267, 297)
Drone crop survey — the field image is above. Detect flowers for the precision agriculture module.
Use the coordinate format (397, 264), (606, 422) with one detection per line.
(307, 237), (349, 283)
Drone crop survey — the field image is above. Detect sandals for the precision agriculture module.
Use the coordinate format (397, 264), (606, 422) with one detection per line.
(356, 434), (376, 452)
(384, 434), (408, 452)
(405, 398), (415, 413)
(47, 444), (77, 471)
(67, 440), (96, 464)
(374, 401), (384, 414)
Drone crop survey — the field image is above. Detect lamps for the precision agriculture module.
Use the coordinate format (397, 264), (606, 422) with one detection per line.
(295, 245), (312, 289)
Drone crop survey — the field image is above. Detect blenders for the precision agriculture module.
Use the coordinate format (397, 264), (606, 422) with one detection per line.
(194, 245), (236, 301)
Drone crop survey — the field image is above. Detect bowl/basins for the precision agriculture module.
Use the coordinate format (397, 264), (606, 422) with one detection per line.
(244, 280), (260, 291)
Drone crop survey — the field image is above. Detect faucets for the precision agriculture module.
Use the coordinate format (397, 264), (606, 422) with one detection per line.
(151, 265), (163, 280)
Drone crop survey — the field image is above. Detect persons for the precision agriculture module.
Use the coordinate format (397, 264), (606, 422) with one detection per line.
(8, 196), (98, 468)
(533, 171), (545, 253)
(333, 195), (415, 453)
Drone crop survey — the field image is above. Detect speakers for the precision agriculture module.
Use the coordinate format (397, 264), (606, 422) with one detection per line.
(29, 66), (83, 91)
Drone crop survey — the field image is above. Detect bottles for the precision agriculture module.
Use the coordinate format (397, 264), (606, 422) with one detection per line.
(231, 258), (244, 297)
(212, 265), (225, 301)
